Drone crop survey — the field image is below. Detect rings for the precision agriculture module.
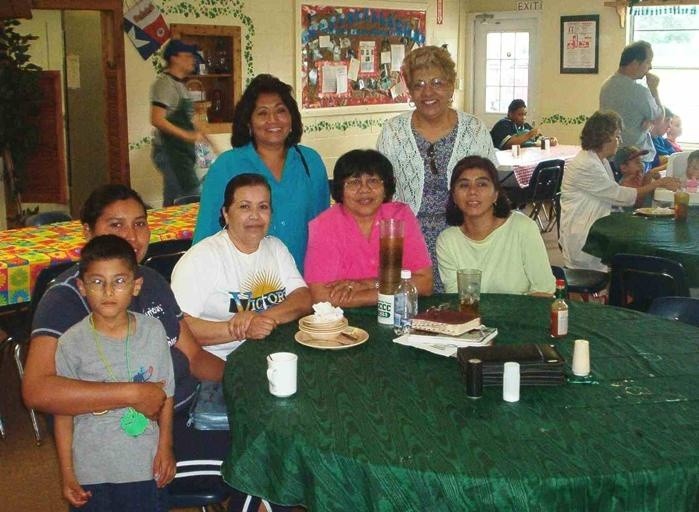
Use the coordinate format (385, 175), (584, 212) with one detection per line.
(347, 285), (352, 291)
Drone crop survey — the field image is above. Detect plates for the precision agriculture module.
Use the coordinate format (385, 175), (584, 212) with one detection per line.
(634, 206), (675, 218)
(294, 326), (369, 351)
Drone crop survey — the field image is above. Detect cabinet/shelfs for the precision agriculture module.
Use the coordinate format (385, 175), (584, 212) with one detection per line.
(169, 24), (242, 134)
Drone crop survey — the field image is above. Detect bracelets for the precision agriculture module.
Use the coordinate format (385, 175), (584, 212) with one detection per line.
(375, 279), (378, 291)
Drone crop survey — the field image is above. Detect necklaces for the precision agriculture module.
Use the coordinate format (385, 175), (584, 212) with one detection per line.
(87, 310), (148, 437)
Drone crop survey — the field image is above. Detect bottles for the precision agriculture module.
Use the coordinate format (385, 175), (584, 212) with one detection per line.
(570, 339), (592, 377)
(550, 280), (570, 338)
(501, 361), (523, 401)
(466, 357), (484, 401)
(392, 270), (419, 336)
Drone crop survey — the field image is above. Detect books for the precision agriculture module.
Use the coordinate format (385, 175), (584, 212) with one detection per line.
(391, 310), (566, 388)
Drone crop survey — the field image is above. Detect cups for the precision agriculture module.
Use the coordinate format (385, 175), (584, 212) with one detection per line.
(456, 269), (482, 314)
(511, 144), (521, 158)
(378, 216), (405, 326)
(264, 353), (299, 399)
(541, 136), (552, 151)
(673, 192), (689, 222)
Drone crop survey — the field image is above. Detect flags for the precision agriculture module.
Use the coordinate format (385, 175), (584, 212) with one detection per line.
(123, 0), (169, 59)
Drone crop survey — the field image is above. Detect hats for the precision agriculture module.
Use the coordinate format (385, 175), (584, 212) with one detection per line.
(613, 145), (649, 172)
(163, 40), (195, 55)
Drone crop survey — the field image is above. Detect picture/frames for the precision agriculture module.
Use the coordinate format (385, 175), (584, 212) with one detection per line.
(560, 15), (599, 74)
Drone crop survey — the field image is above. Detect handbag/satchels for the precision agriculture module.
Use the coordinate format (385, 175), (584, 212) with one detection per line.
(186, 380), (230, 431)
(458, 344), (567, 387)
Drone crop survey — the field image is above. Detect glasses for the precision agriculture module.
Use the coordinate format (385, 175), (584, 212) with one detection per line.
(81, 278), (131, 294)
(344, 177), (383, 191)
(411, 78), (447, 88)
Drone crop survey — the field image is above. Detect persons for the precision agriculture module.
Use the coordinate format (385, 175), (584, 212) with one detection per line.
(434, 156), (557, 297)
(170, 173), (311, 362)
(191, 74), (330, 281)
(376, 45), (500, 295)
(54, 234), (178, 512)
(490, 99), (558, 150)
(560, 40), (699, 308)
(148, 38), (218, 205)
(304, 149), (433, 309)
(22, 185), (279, 512)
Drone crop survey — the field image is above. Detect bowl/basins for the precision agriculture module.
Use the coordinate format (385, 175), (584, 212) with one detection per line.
(297, 316), (348, 340)
